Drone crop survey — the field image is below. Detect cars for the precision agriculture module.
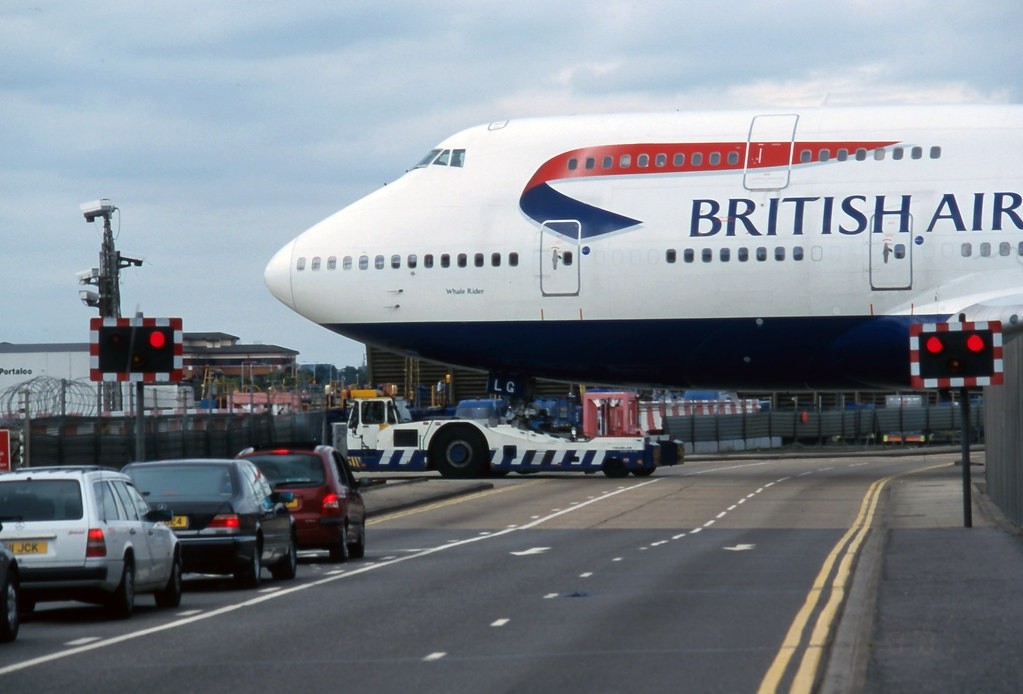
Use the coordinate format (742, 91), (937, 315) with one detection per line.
(0, 548), (24, 640)
(240, 445), (368, 561)
(125, 458), (302, 588)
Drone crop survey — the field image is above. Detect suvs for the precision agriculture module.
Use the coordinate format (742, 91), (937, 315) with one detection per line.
(0, 465), (185, 615)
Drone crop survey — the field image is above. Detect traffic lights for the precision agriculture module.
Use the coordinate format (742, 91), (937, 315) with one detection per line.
(97, 325), (176, 375)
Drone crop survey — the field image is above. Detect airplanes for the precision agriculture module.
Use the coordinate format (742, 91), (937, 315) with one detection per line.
(273, 100), (1023, 398)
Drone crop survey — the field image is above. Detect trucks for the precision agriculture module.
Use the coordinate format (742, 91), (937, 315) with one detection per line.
(339, 394), (687, 483)
